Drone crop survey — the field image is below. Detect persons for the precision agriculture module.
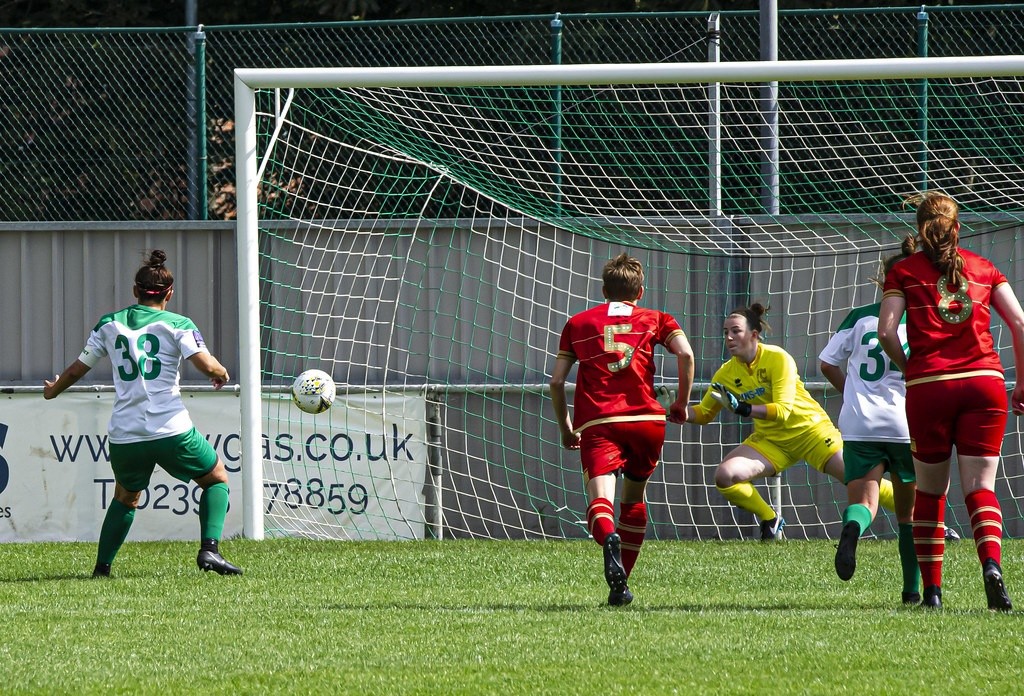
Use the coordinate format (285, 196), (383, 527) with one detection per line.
(42, 249), (243, 580)
(818, 235), (922, 603)
(878, 192), (1024, 611)
(653, 303), (961, 542)
(549, 254), (695, 606)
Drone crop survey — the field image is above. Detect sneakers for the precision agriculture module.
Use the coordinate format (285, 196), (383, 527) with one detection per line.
(944, 528), (960, 541)
(196, 549), (244, 575)
(607, 589), (633, 606)
(983, 558), (1013, 612)
(92, 563), (111, 578)
(902, 591), (921, 605)
(603, 532), (629, 594)
(834, 520), (861, 581)
(760, 512), (786, 543)
(920, 584), (942, 609)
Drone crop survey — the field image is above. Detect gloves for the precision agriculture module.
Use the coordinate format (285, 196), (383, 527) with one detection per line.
(710, 382), (752, 418)
(653, 385), (676, 417)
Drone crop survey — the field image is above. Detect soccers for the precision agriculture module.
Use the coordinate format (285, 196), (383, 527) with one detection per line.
(292, 369), (336, 415)
